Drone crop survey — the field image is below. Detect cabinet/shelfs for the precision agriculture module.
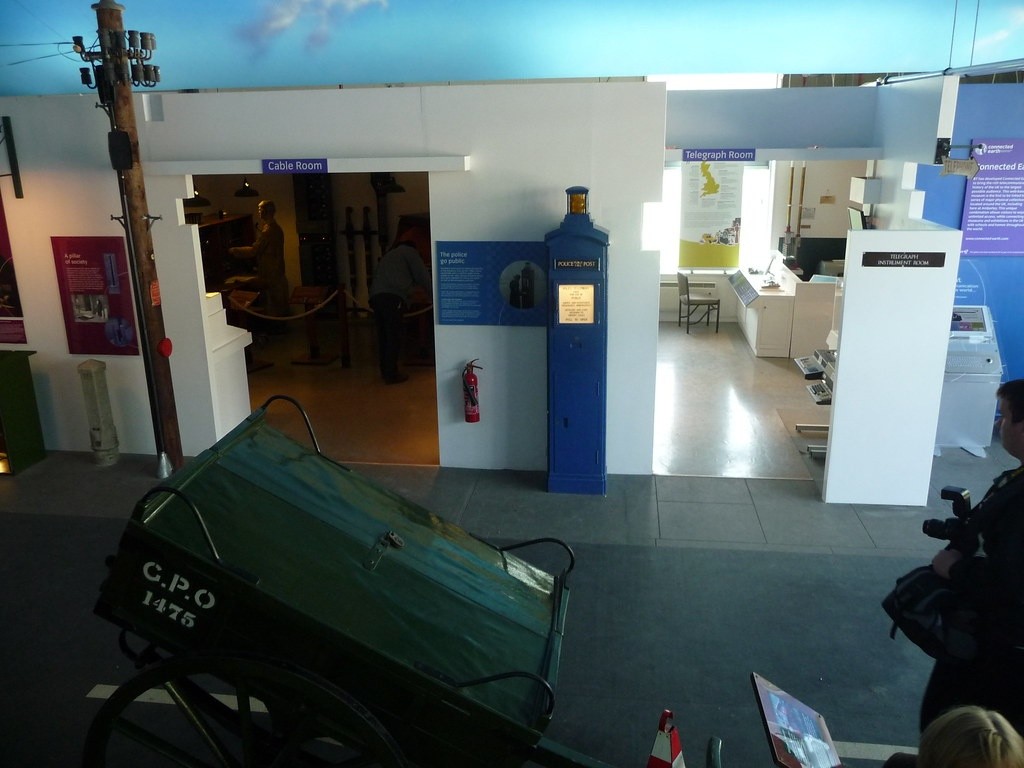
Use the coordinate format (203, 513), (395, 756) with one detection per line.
(196, 212), (254, 289)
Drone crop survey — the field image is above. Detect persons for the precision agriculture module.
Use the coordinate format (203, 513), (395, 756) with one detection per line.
(882, 380), (1024, 734)
(92, 299), (102, 316)
(75, 296), (81, 313)
(367, 239), (432, 385)
(83, 293), (92, 311)
(225, 201), (284, 288)
(883, 706), (1024, 768)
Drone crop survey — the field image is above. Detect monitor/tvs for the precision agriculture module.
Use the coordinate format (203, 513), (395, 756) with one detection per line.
(847, 206), (867, 230)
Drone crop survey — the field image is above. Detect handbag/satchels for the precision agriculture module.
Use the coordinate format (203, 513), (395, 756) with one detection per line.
(882, 565), (991, 664)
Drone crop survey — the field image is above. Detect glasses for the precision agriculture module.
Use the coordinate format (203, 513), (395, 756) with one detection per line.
(994, 410), (1011, 422)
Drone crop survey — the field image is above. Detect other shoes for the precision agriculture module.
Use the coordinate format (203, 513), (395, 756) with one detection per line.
(387, 373), (409, 384)
(271, 326), (288, 335)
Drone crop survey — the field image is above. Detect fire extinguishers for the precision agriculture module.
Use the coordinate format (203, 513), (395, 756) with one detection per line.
(461, 358), (483, 424)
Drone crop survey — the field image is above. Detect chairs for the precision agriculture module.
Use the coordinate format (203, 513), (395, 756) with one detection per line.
(677, 271), (720, 334)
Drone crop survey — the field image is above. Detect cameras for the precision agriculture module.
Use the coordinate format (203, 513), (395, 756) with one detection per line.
(923, 486), (972, 541)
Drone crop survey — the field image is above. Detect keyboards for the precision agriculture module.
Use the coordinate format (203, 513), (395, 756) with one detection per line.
(809, 384), (830, 400)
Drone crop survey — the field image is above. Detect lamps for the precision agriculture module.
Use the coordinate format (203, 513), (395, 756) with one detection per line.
(183, 185), (211, 206)
(379, 177), (406, 193)
(233, 178), (259, 198)
